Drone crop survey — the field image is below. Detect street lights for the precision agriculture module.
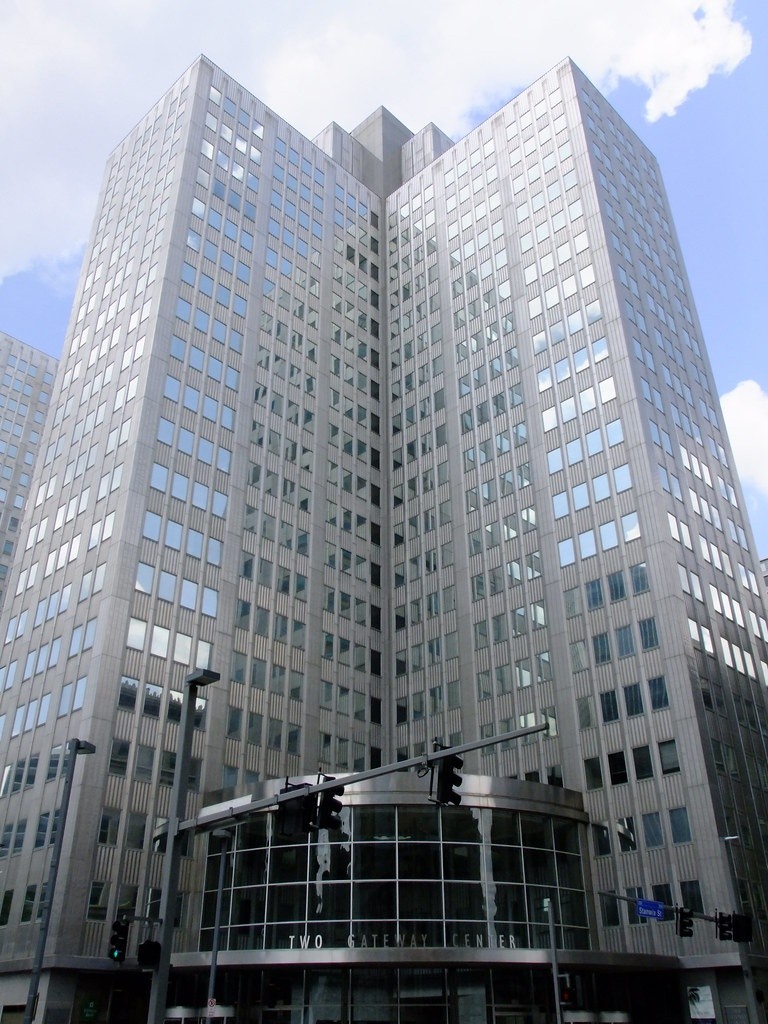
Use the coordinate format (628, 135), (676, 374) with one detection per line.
(144, 670), (221, 1024)
(23, 738), (98, 1024)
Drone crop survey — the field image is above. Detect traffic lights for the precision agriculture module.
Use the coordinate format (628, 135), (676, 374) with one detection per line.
(107, 920), (128, 963)
(436, 747), (464, 806)
(679, 907), (694, 939)
(318, 775), (344, 831)
(719, 912), (732, 940)
